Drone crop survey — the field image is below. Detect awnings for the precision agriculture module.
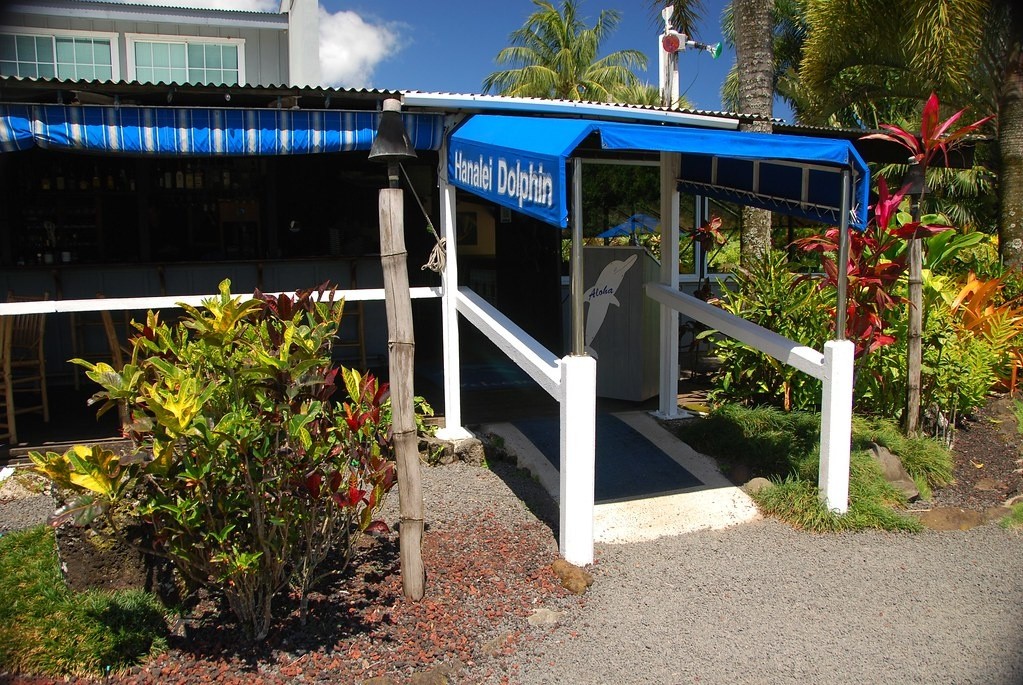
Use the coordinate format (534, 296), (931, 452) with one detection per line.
(0, 100), (446, 153)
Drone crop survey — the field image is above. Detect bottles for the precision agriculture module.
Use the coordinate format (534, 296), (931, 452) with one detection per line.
(13, 155), (258, 267)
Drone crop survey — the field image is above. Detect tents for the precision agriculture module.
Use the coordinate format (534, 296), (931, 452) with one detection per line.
(445, 114), (870, 355)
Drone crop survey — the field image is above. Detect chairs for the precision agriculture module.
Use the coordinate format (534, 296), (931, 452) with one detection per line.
(0, 289), (49, 444)
(53, 269), (368, 427)
